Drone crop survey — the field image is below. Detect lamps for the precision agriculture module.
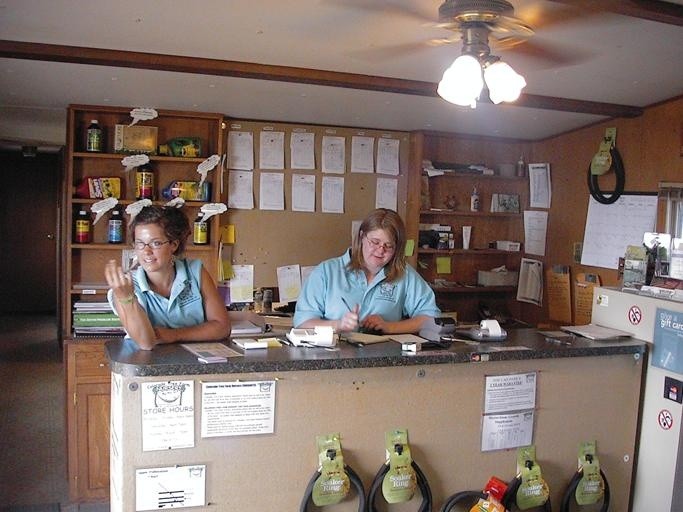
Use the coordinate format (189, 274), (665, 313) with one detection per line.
(437, 1), (533, 108)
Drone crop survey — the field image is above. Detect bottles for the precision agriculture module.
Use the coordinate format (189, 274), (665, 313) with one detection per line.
(73, 175), (122, 200)
(192, 212), (209, 245)
(470, 185), (480, 212)
(106, 210), (123, 244)
(517, 154), (526, 177)
(74, 210), (89, 244)
(135, 162), (156, 201)
(253, 288), (272, 314)
(85, 119), (104, 154)
(160, 179), (212, 202)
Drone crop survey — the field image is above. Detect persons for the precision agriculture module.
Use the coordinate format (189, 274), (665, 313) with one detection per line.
(101, 203), (232, 352)
(290, 206), (442, 339)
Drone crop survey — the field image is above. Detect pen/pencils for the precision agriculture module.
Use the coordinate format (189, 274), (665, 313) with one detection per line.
(442, 337), (481, 345)
(341, 297), (360, 324)
(545, 337), (572, 345)
(278, 339), (291, 346)
(347, 342), (363, 348)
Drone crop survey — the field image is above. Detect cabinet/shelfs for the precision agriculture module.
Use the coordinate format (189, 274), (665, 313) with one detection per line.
(418, 168), (520, 298)
(68, 101), (219, 337)
(63, 337), (112, 512)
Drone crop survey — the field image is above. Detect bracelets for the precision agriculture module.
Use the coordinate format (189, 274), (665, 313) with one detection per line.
(116, 295), (137, 305)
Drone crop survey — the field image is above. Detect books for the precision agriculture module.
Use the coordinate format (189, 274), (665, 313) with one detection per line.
(72, 298), (128, 338)
(419, 222), (455, 250)
(335, 328), (389, 347)
(560, 319), (635, 344)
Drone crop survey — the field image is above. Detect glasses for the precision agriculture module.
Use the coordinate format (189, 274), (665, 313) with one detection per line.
(132, 240), (169, 250)
(365, 232), (396, 252)
